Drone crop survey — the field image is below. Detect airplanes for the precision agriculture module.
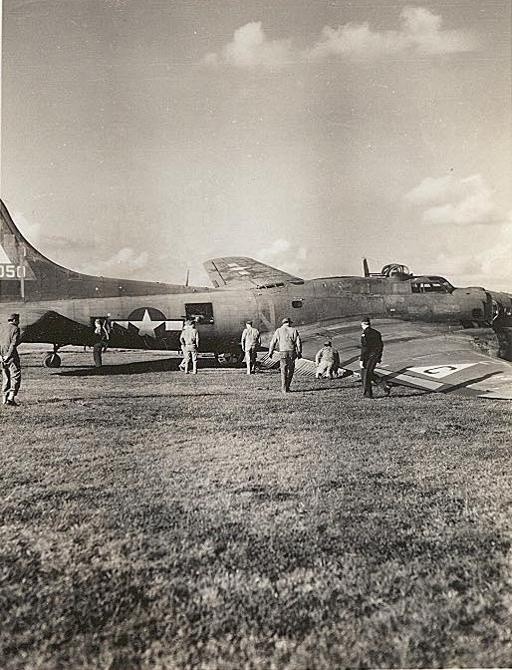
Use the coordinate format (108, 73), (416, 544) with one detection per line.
(0, 201), (512, 402)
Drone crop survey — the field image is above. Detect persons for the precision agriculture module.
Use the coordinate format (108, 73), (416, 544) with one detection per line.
(241, 320), (261, 375)
(94, 319), (105, 367)
(179, 320), (200, 374)
(316, 341), (340, 379)
(360, 318), (384, 398)
(268, 317), (302, 392)
(0, 313), (21, 406)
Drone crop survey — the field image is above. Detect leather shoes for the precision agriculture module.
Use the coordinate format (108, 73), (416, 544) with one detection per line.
(361, 394), (375, 400)
(385, 386), (391, 396)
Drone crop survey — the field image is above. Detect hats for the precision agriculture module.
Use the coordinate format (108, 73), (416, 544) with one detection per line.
(94, 318), (101, 323)
(281, 317), (294, 323)
(362, 318), (370, 322)
(323, 341), (332, 346)
(184, 320), (196, 325)
(246, 320), (254, 324)
(8, 313), (20, 320)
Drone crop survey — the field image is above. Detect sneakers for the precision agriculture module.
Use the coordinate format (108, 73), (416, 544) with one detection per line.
(178, 365), (198, 375)
(2, 397), (19, 405)
